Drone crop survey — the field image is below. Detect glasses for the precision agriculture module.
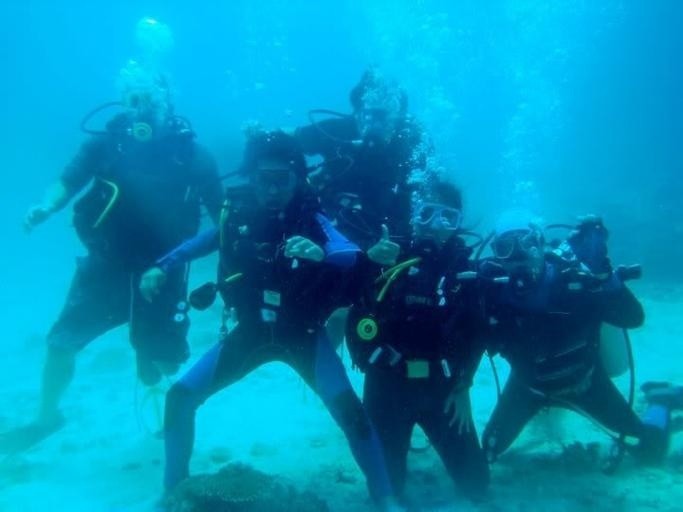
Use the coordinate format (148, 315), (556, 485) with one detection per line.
(124, 92), (163, 114)
(361, 86), (404, 109)
(492, 232), (540, 258)
(251, 169), (297, 192)
(416, 204), (462, 229)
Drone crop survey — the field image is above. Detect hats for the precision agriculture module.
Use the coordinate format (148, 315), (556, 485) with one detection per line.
(493, 212), (546, 244)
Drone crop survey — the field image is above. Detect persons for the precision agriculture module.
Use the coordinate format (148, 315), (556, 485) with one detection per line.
(342, 177), (489, 505)
(138, 127), (402, 512)
(7, 73), (225, 450)
(475, 210), (671, 469)
(283, 74), (431, 349)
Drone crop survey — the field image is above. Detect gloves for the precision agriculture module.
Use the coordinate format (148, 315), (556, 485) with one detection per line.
(570, 216), (608, 273)
